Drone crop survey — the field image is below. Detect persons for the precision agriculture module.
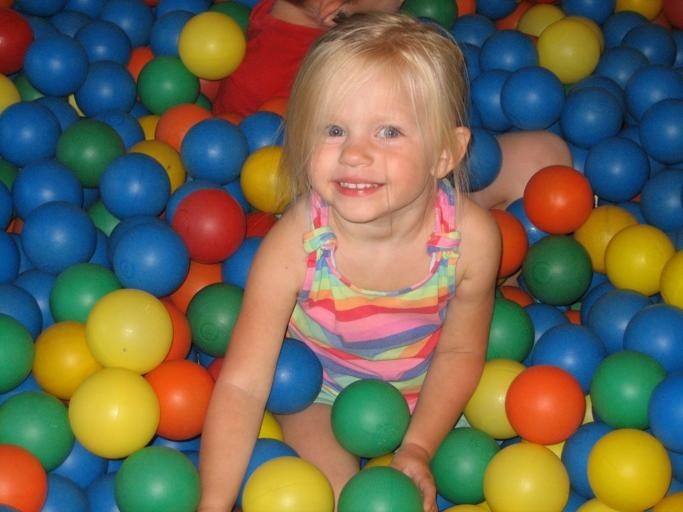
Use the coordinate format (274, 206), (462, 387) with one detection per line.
(210, 1), (574, 210)
(197, 10), (500, 512)
